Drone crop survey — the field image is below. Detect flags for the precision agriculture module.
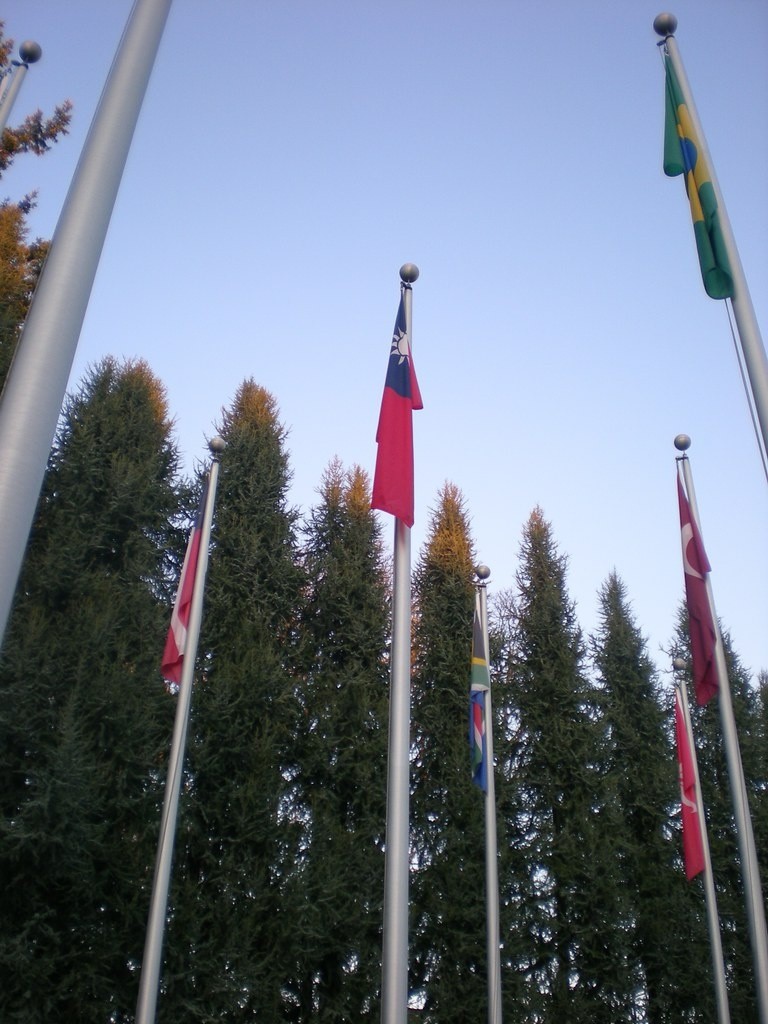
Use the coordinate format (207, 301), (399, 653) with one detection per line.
(664, 57), (739, 302)
(676, 461), (720, 707)
(675, 703), (706, 881)
(161, 475), (208, 686)
(369, 288), (426, 528)
(469, 591), (492, 792)
